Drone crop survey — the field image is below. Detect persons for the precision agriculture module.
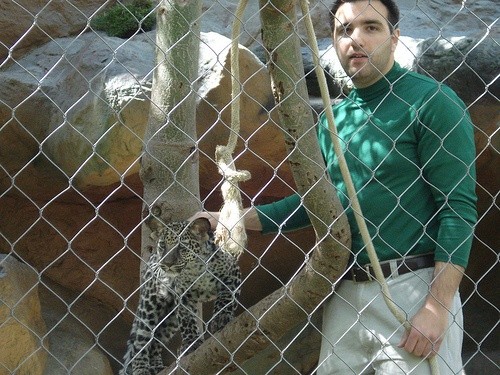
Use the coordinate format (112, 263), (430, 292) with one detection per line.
(187, 0), (477, 375)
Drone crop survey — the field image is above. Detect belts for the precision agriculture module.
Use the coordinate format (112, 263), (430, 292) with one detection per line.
(341, 255), (435, 283)
